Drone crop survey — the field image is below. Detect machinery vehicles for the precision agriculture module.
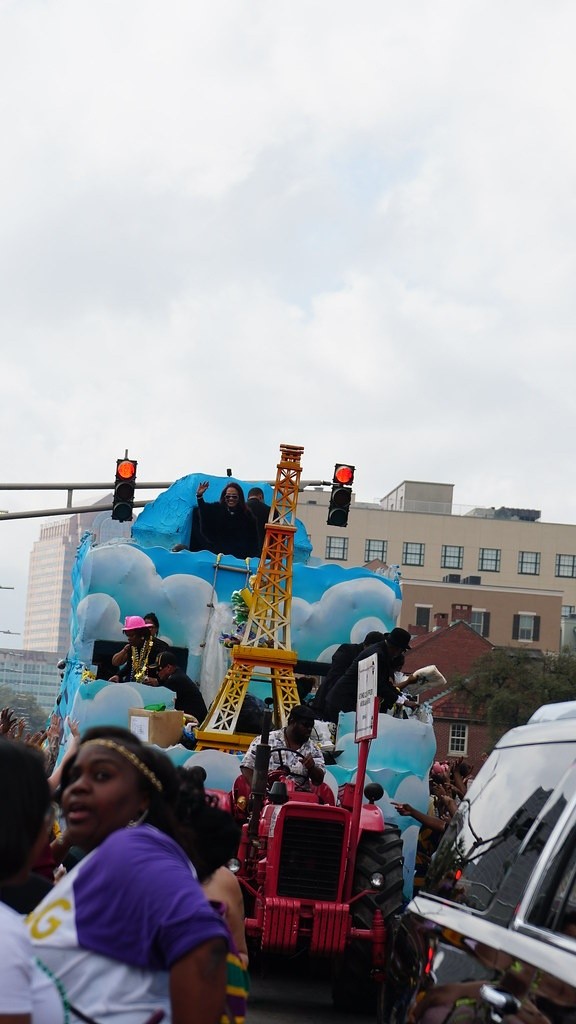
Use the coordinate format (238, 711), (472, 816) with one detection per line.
(51, 442), (442, 1014)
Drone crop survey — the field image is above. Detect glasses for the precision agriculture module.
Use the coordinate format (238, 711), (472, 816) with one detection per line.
(156, 663), (169, 672)
(224, 495), (239, 499)
(293, 716), (314, 727)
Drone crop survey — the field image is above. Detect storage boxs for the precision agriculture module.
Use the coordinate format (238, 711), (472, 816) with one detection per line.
(126, 705), (185, 750)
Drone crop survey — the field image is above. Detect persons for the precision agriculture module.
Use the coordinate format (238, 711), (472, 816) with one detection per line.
(112, 612), (159, 665)
(194, 481), (260, 560)
(245, 486), (280, 558)
(388, 752), (488, 881)
(294, 626), (421, 742)
(239, 705), (325, 805)
(0, 705), (249, 1024)
(107, 615), (170, 684)
(143, 651), (207, 728)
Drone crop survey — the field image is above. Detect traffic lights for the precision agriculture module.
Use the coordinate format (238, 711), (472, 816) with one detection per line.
(111, 460), (137, 521)
(328, 463), (355, 526)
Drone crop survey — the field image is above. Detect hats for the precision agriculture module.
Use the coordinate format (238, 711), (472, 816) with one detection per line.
(122, 616), (153, 630)
(384, 628), (411, 649)
(148, 651), (177, 669)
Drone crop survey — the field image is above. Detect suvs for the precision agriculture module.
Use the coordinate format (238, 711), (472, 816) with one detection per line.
(387, 700), (576, 1024)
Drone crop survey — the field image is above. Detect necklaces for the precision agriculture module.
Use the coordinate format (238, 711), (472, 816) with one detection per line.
(130, 636), (153, 682)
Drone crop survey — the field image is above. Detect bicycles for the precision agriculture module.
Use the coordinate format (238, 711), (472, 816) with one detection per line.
(442, 982), (528, 1024)
